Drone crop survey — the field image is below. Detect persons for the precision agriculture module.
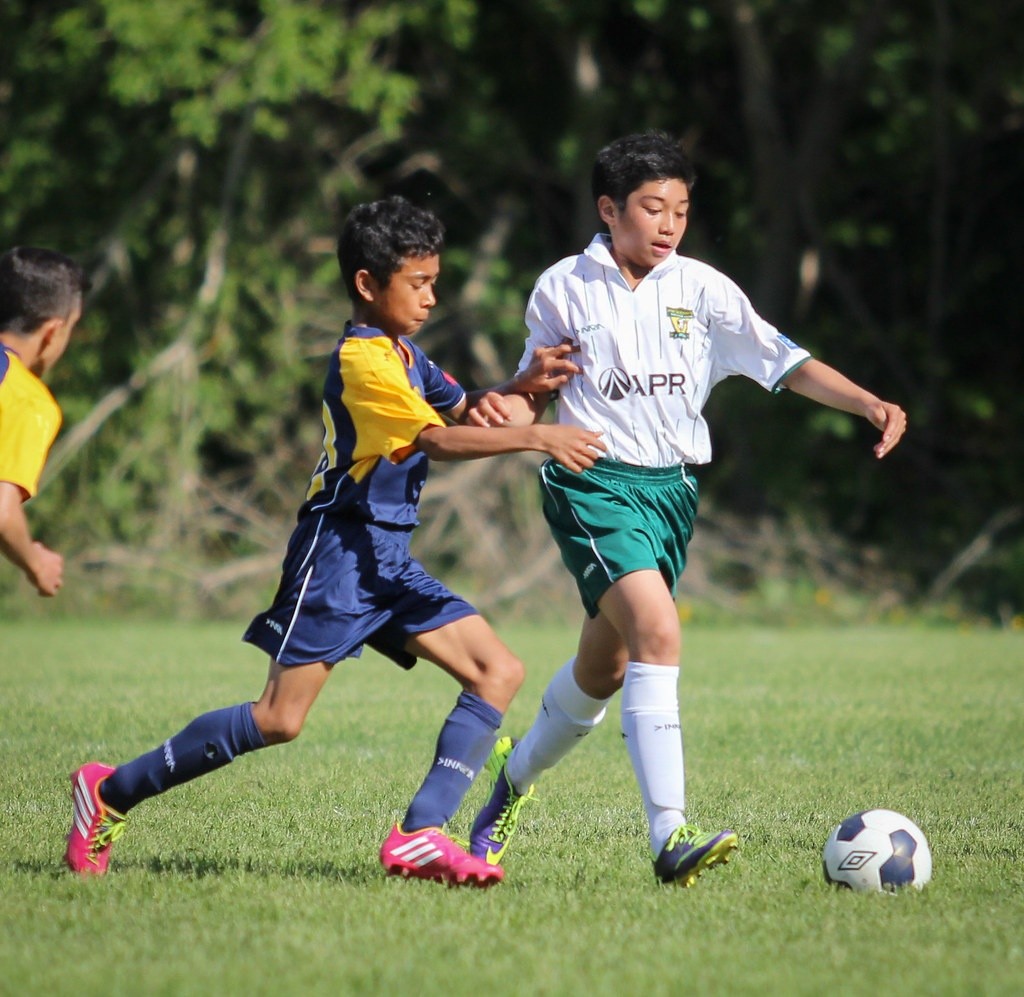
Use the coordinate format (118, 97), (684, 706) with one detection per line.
(0, 245), (92, 599)
(464, 132), (909, 888)
(64, 200), (606, 891)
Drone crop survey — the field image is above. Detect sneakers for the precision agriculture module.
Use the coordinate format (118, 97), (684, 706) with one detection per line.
(63, 763), (127, 878)
(471, 736), (534, 867)
(649, 824), (738, 889)
(380, 823), (504, 889)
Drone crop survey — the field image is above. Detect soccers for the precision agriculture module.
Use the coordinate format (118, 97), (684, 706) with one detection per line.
(821, 809), (934, 899)
